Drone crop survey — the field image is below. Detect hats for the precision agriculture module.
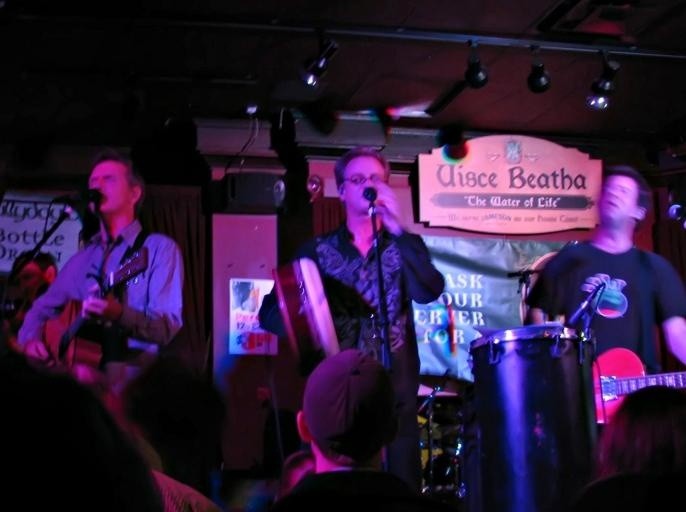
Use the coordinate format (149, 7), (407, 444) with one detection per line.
(304, 350), (396, 457)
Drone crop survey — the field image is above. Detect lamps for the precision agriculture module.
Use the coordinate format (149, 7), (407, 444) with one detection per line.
(585, 44), (626, 112)
(527, 44), (550, 93)
(302, 27), (338, 90)
(466, 35), (488, 88)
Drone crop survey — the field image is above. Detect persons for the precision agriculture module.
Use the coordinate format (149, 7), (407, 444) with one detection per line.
(0, 349), (224, 511)
(258, 149), (445, 495)
(0, 251), (77, 354)
(246, 348), (454, 512)
(15, 155), (183, 397)
(568, 384), (685, 511)
(523, 166), (686, 431)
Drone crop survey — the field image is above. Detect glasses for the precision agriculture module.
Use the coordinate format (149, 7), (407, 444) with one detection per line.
(342, 174), (385, 185)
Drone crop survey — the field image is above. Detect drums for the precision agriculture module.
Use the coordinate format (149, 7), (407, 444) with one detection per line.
(272, 258), (341, 371)
(470, 322), (599, 512)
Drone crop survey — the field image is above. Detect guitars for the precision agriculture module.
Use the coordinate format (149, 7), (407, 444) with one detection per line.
(41, 247), (149, 372)
(592, 348), (686, 425)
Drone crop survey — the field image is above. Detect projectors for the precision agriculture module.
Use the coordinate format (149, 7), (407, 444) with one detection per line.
(298, 38), (375, 136)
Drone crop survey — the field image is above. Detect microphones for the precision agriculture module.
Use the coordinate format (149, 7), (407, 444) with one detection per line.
(667, 204), (686, 229)
(53, 188), (103, 205)
(568, 282), (607, 326)
(361, 187), (378, 219)
